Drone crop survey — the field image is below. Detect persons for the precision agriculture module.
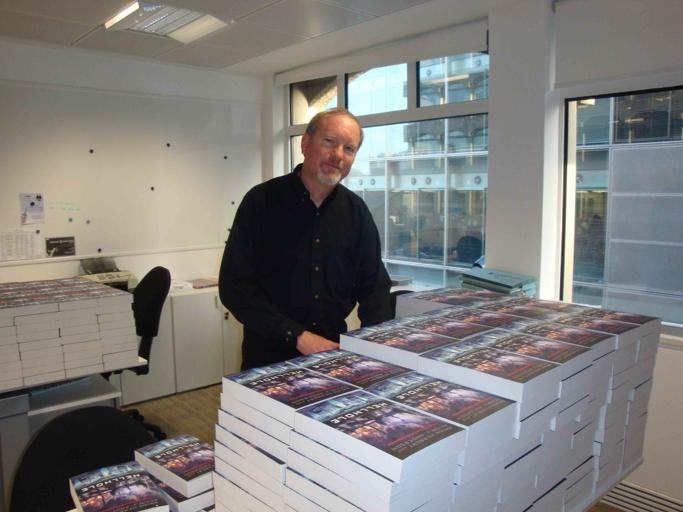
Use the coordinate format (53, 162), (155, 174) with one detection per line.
(216, 105), (395, 372)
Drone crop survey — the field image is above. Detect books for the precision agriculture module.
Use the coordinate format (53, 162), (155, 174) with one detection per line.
(215, 285), (663, 511)
(68, 427), (214, 512)
(0, 274), (140, 395)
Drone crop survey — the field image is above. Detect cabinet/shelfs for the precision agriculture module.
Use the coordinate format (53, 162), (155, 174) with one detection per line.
(109, 287), (244, 406)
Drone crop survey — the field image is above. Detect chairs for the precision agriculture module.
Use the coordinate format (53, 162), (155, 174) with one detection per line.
(4, 406), (158, 512)
(449, 235), (483, 268)
